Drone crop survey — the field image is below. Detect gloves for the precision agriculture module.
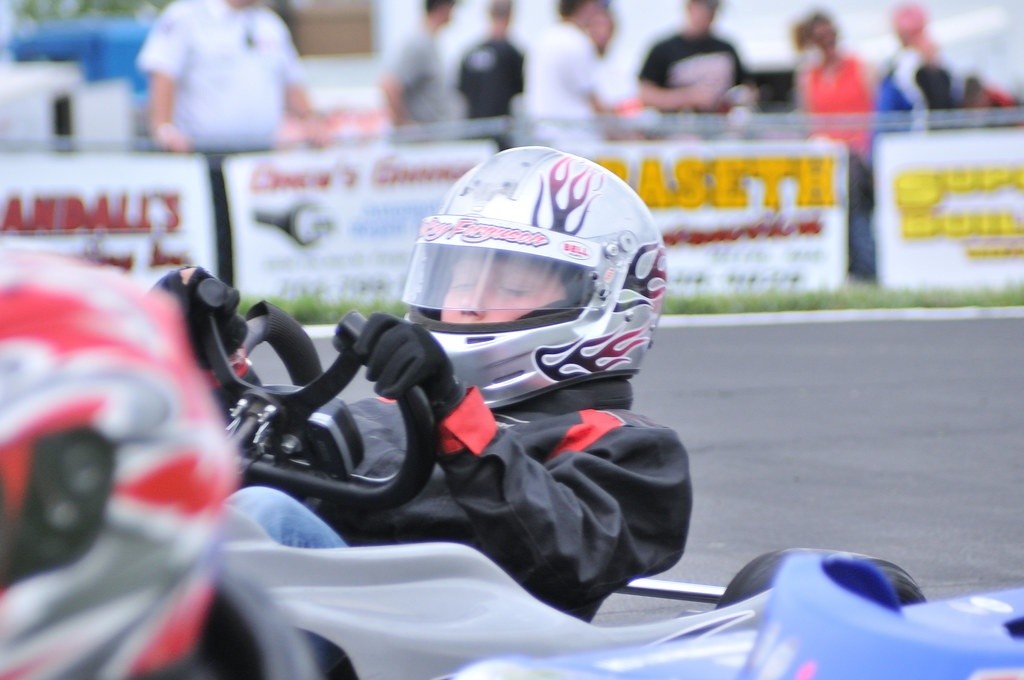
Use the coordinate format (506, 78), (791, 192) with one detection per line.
(331, 311), (467, 420)
(145, 263), (250, 371)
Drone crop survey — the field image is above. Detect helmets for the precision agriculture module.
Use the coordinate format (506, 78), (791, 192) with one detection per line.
(403, 145), (670, 410)
(1, 252), (246, 679)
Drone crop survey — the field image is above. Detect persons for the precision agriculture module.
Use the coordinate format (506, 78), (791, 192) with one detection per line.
(638, 0), (761, 113)
(381, 0), (455, 126)
(138, 145), (692, 620)
(134, 0), (333, 153)
(796, 14), (875, 277)
(525, 0), (614, 116)
(867, 6), (950, 172)
(459, 1), (524, 118)
(0, 234), (244, 680)
